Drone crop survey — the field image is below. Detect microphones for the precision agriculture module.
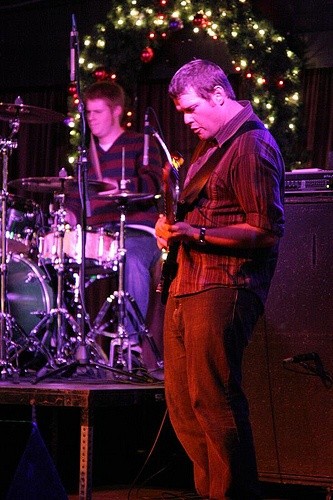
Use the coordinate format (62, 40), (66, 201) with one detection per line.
(143, 112), (150, 164)
(284, 352), (320, 364)
(70, 31), (77, 82)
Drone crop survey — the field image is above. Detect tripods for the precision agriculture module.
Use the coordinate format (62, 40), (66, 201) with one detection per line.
(0, 27), (161, 383)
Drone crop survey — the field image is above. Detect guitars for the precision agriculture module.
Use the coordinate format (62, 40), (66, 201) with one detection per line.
(156, 151), (185, 306)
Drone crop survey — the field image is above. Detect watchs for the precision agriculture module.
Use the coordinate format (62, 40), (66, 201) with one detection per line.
(197, 227), (206, 246)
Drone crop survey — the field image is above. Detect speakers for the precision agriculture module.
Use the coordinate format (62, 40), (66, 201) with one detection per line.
(236, 193), (333, 486)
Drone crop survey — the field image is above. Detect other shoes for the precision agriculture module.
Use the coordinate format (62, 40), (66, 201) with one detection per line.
(114, 352), (140, 371)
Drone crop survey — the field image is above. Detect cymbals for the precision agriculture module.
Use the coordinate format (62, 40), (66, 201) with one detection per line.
(6, 176), (117, 192)
(0, 102), (66, 124)
(90, 188), (152, 204)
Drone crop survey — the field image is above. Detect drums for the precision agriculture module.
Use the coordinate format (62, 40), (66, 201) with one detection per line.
(38, 223), (120, 279)
(0, 251), (77, 371)
(0, 192), (43, 258)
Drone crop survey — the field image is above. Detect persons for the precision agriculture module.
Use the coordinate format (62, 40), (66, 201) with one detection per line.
(156, 64), (286, 500)
(57, 80), (179, 370)
